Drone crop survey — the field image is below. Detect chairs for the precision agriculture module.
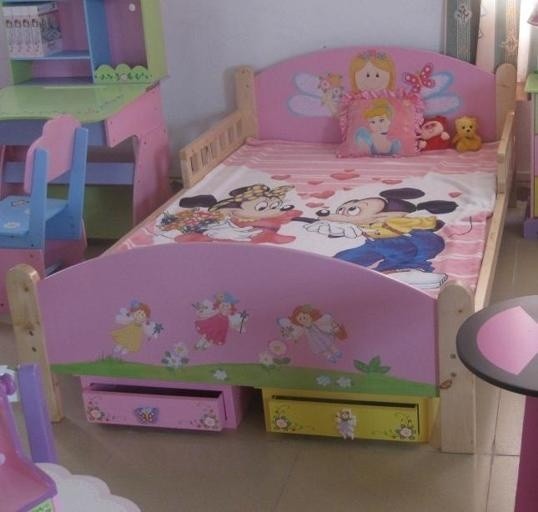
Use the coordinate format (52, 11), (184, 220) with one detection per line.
(0, 113), (88, 314)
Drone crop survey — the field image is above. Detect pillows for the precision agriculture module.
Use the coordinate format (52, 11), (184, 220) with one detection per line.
(335, 87), (425, 158)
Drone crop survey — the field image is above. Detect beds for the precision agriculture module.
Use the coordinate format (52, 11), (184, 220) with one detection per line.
(5, 45), (517, 455)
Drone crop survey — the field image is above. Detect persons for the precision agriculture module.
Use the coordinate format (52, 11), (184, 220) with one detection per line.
(351, 96), (401, 156)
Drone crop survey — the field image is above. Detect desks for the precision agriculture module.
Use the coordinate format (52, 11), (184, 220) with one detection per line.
(456, 293), (538, 512)
(0, 0), (173, 240)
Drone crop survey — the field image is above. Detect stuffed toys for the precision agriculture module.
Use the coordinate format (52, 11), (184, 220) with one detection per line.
(416, 115), (451, 151)
(446, 115), (484, 154)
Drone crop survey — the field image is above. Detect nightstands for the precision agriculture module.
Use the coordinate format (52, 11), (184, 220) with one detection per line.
(523, 72), (538, 238)
